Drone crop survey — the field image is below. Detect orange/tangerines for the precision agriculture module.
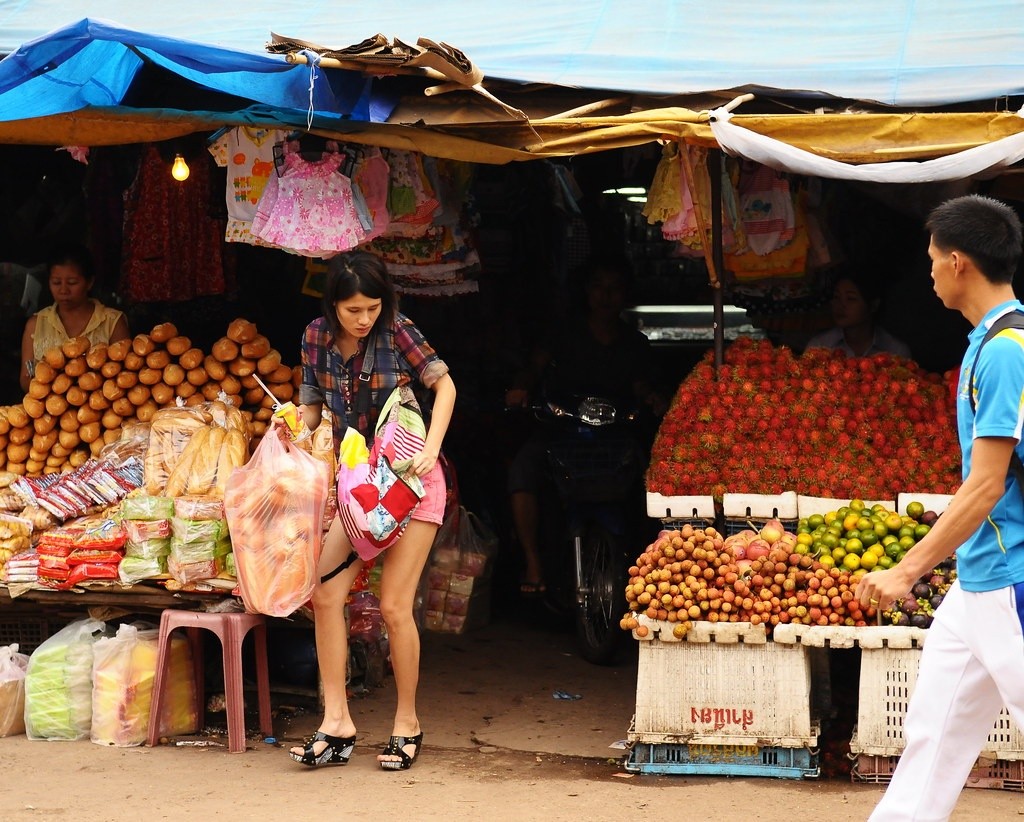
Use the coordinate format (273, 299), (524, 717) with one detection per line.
(795, 500), (931, 577)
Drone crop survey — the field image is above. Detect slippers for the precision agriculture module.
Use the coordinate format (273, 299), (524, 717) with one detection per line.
(519, 579), (545, 597)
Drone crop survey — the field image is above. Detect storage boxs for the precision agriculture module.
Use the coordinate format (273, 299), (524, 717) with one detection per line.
(624, 638), (1024, 791)
(0, 616), (64, 656)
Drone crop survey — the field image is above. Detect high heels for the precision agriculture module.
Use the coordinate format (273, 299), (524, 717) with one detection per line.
(289, 730), (356, 766)
(379, 732), (423, 771)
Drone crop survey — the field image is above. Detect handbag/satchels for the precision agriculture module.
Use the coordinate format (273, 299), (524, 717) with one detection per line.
(368, 386), (427, 468)
(224, 417), (328, 618)
(336, 425), (426, 562)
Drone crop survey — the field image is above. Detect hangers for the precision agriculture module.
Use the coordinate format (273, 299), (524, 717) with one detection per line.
(273, 136), (364, 179)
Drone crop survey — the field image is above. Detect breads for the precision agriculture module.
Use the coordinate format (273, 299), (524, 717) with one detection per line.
(0, 319), (325, 618)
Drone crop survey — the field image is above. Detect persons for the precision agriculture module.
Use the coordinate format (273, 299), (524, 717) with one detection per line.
(848, 191), (1023, 795)
(502, 251), (671, 601)
(267, 250), (458, 771)
(804, 271), (913, 360)
(20, 243), (130, 395)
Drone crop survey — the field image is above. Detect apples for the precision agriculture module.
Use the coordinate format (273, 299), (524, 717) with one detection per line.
(646, 520), (797, 574)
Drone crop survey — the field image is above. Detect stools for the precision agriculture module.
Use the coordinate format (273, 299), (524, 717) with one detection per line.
(145, 609), (273, 754)
